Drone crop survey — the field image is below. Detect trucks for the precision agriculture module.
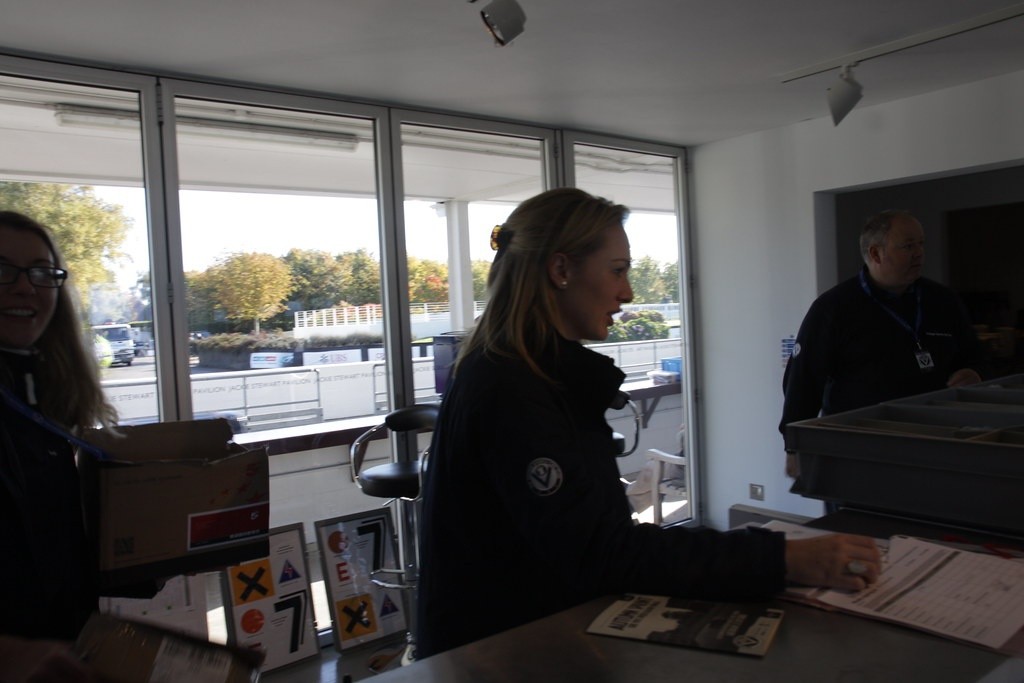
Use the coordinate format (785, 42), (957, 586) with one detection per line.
(90, 322), (135, 366)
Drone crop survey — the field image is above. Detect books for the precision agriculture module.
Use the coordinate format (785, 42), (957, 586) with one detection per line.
(585, 592), (786, 658)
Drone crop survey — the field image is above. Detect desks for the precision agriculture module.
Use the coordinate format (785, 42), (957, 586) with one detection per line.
(360, 519), (1024, 683)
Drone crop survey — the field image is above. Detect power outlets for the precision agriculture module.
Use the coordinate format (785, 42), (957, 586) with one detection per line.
(750, 483), (764, 502)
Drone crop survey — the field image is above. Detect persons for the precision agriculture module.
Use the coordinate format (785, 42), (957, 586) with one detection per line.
(410, 188), (881, 657)
(0, 208), (174, 683)
(776, 209), (981, 479)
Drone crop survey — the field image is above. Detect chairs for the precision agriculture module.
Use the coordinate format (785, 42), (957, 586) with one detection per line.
(646, 449), (688, 525)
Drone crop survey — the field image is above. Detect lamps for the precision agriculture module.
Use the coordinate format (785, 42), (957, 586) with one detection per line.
(826, 70), (863, 127)
(479, 0), (526, 47)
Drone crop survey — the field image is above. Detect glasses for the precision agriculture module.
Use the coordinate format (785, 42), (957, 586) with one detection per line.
(0, 262), (68, 289)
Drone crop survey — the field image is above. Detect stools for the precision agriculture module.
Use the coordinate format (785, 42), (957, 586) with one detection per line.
(611, 390), (642, 525)
(350, 404), (441, 674)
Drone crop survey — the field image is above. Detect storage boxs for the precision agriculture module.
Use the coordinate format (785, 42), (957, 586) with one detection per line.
(648, 370), (676, 385)
(73, 611), (267, 683)
(661, 358), (681, 381)
(78, 417), (270, 589)
